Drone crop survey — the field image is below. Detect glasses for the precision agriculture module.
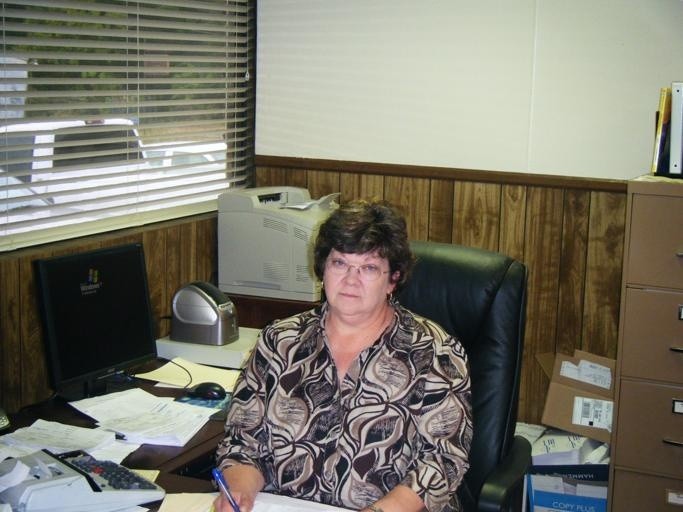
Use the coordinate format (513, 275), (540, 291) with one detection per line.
(328, 257), (393, 281)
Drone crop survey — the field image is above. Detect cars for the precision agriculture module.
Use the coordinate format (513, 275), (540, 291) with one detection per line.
(142, 141), (229, 212)
(0, 167), (114, 248)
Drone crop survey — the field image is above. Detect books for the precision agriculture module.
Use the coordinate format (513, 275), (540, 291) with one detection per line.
(649, 87), (673, 175)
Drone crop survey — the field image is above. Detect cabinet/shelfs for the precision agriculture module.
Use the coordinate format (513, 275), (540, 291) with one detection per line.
(608, 173), (683, 512)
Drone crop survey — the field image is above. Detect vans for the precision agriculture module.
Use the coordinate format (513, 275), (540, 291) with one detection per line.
(0, 118), (153, 217)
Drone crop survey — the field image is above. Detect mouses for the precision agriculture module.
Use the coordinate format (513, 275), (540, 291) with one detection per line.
(187, 382), (226, 401)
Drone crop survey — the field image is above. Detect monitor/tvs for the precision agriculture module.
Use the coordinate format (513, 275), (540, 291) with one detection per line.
(34, 243), (157, 393)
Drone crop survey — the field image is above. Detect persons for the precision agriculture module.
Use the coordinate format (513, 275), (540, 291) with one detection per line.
(210, 197), (475, 511)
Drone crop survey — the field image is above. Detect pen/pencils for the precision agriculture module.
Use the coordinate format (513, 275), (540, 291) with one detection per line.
(212, 468), (241, 512)
(96, 370), (125, 381)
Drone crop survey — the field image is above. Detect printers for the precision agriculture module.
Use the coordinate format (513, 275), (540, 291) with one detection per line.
(218, 185), (338, 303)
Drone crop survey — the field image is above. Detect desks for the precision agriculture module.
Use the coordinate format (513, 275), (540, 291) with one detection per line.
(223, 292), (325, 330)
(1, 330), (335, 512)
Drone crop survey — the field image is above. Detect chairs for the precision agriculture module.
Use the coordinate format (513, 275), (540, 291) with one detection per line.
(397, 240), (532, 512)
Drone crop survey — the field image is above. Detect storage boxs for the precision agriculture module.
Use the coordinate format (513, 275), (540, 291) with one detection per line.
(533, 350), (617, 443)
(514, 422), (610, 512)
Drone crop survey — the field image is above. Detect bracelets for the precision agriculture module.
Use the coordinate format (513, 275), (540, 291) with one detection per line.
(366, 504), (383, 512)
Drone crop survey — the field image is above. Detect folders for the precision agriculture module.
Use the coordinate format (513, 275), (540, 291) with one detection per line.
(668, 82), (683, 174)
